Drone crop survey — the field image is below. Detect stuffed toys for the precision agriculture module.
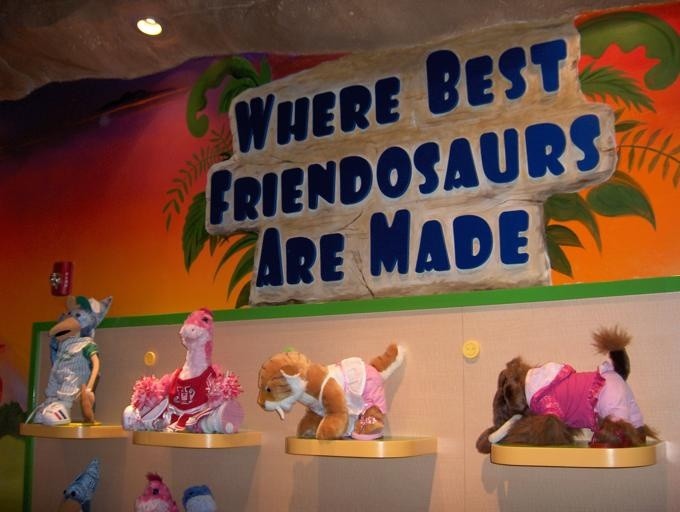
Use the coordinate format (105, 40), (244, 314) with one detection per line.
(27, 293), (114, 425)
(118, 306), (246, 436)
(473, 321), (658, 454)
(132, 470), (178, 511)
(181, 484), (217, 512)
(255, 340), (406, 441)
(60, 457), (102, 511)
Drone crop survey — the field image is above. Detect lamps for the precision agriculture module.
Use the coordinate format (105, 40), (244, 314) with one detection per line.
(136, 15), (164, 35)
